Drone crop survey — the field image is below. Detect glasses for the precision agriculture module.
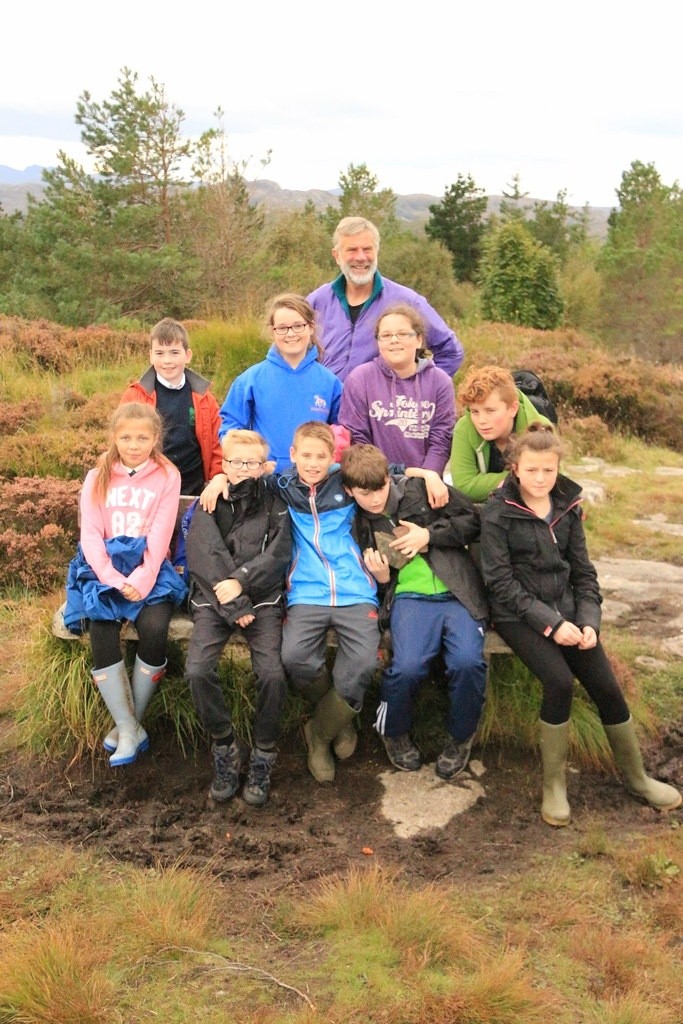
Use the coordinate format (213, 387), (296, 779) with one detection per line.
(273, 323), (309, 335)
(378, 328), (416, 343)
(225, 459), (264, 471)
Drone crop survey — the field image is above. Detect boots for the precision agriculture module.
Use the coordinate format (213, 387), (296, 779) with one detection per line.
(90, 659), (150, 767)
(300, 666), (357, 762)
(537, 716), (572, 826)
(104, 654), (168, 753)
(603, 713), (682, 811)
(303, 688), (363, 785)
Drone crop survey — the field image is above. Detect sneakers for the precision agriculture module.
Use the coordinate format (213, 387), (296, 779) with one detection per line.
(210, 734), (247, 803)
(379, 732), (421, 772)
(435, 732), (475, 779)
(243, 748), (279, 805)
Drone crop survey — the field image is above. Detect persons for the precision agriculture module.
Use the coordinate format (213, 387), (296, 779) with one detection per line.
(64, 404), (190, 769)
(479, 421), (683, 825)
(449, 365), (559, 504)
(341, 442), (488, 780)
(338, 303), (457, 478)
(182, 428), (295, 804)
(199, 421), (450, 785)
(300, 216), (465, 383)
(219, 294), (344, 477)
(119, 317), (224, 496)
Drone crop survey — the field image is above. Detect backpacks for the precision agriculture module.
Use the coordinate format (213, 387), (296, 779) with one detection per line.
(512, 370), (559, 423)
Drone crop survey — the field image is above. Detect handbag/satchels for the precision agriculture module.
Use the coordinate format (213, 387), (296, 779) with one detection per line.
(173, 495), (200, 582)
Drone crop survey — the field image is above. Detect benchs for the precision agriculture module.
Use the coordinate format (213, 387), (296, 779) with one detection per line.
(53, 494), (516, 747)
